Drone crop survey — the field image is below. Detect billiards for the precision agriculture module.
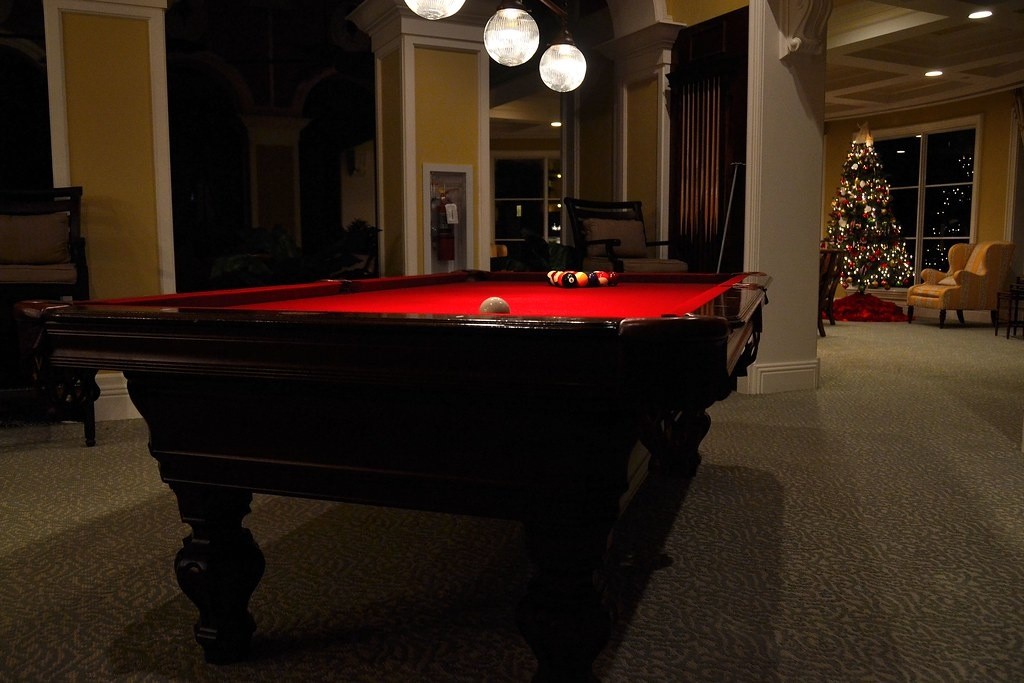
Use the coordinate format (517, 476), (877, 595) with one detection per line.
(478, 296), (511, 316)
(546, 268), (621, 290)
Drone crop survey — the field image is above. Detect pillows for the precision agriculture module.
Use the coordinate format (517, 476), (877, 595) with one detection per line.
(0, 213), (70, 265)
(584, 218), (649, 259)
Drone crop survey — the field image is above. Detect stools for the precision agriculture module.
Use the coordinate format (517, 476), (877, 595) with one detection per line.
(0, 297), (96, 446)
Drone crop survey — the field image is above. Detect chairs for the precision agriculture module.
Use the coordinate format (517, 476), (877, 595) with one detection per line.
(0, 187), (88, 298)
(906, 241), (1016, 328)
(818, 249), (844, 337)
(564, 197), (687, 274)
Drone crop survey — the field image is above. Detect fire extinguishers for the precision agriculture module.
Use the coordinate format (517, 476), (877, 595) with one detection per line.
(429, 188), (459, 264)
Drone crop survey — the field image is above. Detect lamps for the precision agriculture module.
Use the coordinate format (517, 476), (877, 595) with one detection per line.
(404, 0), (465, 20)
(538, 19), (586, 93)
(484, 0), (539, 66)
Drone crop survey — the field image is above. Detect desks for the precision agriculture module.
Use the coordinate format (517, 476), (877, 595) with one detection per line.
(38, 269), (773, 683)
